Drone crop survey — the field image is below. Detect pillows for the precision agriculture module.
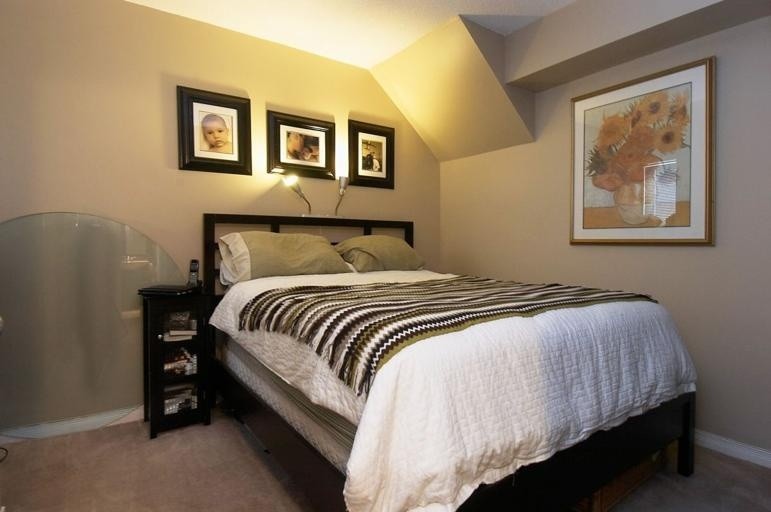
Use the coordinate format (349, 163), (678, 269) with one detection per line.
(218, 230), (427, 286)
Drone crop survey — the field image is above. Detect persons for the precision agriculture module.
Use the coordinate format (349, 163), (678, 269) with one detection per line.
(288, 143), (316, 163)
(365, 149), (383, 173)
(195, 110), (234, 154)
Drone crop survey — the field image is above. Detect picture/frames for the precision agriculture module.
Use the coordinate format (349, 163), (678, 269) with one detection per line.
(570, 54), (717, 247)
(176, 83), (396, 191)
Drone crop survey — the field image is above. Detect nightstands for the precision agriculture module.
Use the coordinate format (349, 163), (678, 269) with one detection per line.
(142, 285), (215, 438)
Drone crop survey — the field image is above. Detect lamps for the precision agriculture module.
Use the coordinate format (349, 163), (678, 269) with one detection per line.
(286, 168), (352, 219)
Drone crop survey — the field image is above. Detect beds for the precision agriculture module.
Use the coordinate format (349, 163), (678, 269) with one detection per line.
(202, 212), (697, 512)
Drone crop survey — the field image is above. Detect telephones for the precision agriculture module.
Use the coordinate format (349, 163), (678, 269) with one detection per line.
(185, 258), (203, 289)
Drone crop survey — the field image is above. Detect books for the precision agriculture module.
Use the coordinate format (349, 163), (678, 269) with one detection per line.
(161, 311), (201, 418)
